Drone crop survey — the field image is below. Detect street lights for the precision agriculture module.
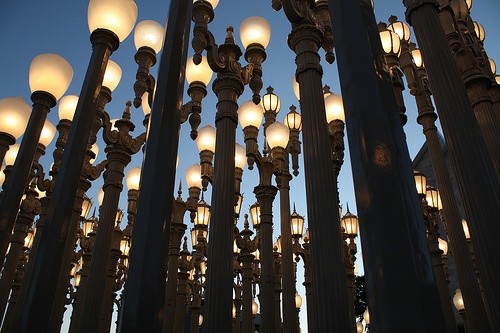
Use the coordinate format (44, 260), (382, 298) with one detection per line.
(0, 0), (500, 333)
